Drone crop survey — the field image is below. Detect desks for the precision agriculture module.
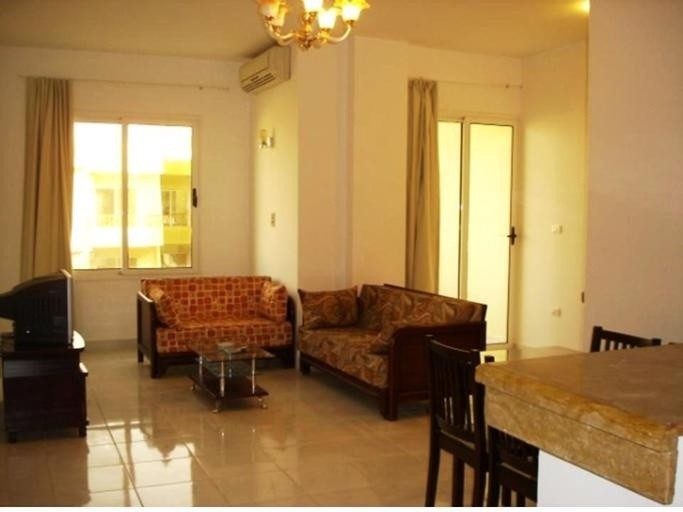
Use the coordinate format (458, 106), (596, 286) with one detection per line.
(480, 346), (580, 364)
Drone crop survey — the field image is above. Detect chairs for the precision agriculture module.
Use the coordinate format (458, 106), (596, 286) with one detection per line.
(589, 325), (662, 353)
(424, 334), (490, 508)
(486, 355), (538, 506)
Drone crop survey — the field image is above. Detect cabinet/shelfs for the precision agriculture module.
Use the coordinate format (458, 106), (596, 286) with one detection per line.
(474, 345), (683, 506)
(0, 332), (89, 443)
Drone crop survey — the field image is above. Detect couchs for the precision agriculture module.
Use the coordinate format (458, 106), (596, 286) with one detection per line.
(296, 283), (488, 421)
(136, 274), (298, 379)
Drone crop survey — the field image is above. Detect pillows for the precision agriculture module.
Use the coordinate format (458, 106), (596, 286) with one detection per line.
(298, 287), (361, 330)
(147, 282), (180, 328)
(258, 282), (289, 324)
(366, 320), (434, 356)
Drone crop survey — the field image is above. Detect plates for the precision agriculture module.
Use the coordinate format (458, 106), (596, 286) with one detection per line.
(217, 340), (247, 354)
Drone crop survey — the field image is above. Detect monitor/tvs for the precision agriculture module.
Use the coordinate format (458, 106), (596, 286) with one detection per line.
(0, 267), (73, 350)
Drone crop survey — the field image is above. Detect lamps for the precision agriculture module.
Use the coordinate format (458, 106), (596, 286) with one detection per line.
(256, 1), (371, 52)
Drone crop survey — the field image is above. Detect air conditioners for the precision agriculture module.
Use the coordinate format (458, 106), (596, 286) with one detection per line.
(239, 46), (293, 97)
